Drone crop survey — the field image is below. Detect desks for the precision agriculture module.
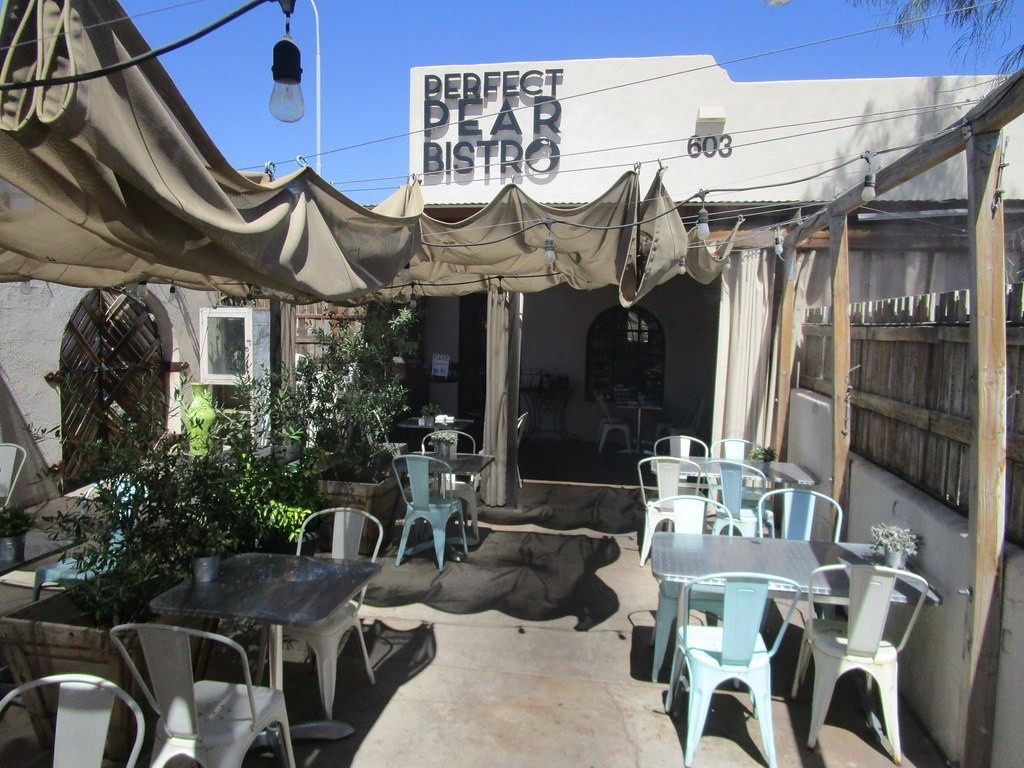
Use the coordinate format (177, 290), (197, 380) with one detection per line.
(614, 387), (664, 454)
(149, 552), (382, 690)
(651, 455), (819, 513)
(392, 451), (495, 496)
(398, 417), (474, 454)
(651, 532), (943, 686)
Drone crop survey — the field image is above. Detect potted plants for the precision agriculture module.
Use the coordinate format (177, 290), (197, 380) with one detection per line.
(0, 356), (312, 760)
(235, 290), (424, 554)
(747, 444), (780, 476)
(421, 402), (442, 426)
(871, 521), (924, 570)
(427, 432), (458, 457)
(154, 456), (244, 582)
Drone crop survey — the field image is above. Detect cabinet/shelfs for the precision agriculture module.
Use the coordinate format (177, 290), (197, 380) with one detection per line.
(199, 307), (270, 447)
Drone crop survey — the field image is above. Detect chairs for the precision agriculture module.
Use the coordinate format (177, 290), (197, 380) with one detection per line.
(0, 413), (529, 768)
(593, 389), (929, 768)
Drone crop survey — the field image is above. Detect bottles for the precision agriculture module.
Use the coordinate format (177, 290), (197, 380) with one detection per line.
(185, 382), (216, 456)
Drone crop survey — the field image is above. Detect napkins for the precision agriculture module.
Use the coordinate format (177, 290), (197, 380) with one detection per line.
(435, 415), (455, 423)
